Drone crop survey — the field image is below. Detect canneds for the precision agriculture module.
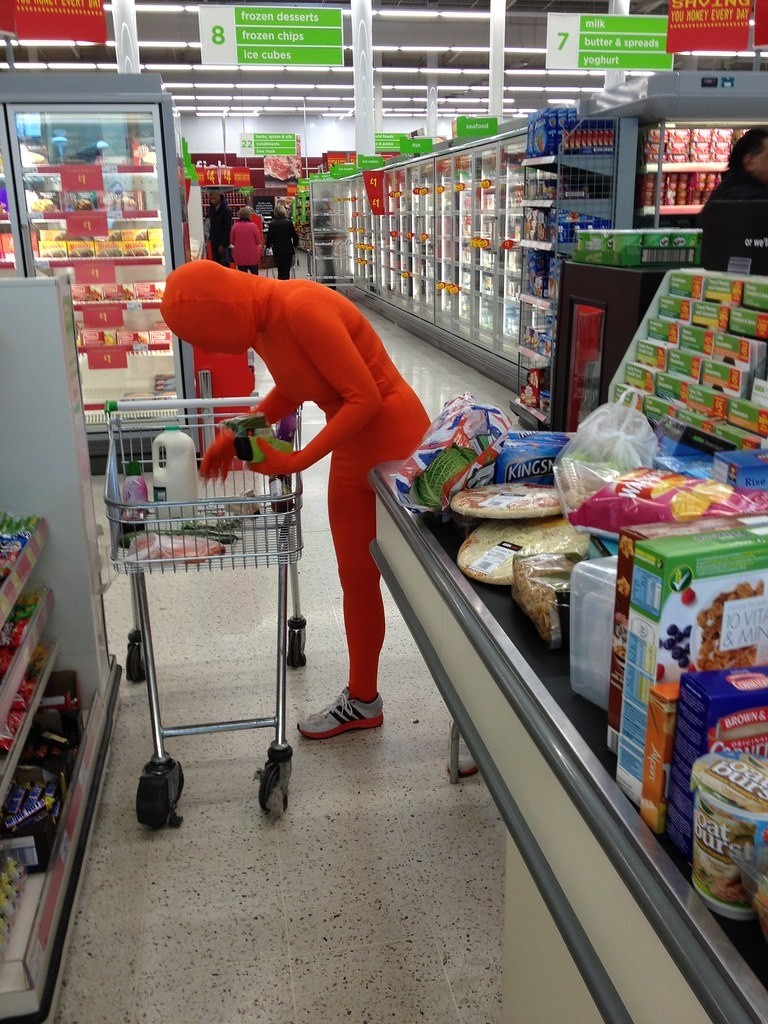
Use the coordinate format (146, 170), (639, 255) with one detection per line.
(641, 128), (750, 207)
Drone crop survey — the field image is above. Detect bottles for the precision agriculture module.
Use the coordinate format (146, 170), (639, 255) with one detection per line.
(558, 226), (564, 243)
(122, 461), (149, 515)
(573, 226), (579, 242)
(153, 426), (199, 519)
(549, 276), (553, 299)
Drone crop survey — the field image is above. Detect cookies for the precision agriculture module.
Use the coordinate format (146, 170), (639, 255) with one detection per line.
(452, 485), (591, 584)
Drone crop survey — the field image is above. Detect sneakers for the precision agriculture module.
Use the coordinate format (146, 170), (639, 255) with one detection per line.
(448, 720), (479, 777)
(297, 687), (384, 740)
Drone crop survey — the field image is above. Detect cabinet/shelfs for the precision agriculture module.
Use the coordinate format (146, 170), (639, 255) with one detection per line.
(193, 149), (344, 269)
(509, 116), (639, 431)
(0, 273), (124, 1024)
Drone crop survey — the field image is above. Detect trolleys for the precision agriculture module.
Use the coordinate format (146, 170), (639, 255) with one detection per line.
(104, 396), (305, 830)
(263, 246), (298, 279)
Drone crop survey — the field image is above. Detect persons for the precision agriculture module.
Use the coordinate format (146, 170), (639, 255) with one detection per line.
(229, 206), (265, 275)
(265, 205), (298, 280)
(159, 259), (479, 777)
(697, 127), (768, 277)
(209, 190), (232, 266)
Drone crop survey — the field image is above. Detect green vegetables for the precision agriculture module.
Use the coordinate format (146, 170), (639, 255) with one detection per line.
(119, 530), (240, 544)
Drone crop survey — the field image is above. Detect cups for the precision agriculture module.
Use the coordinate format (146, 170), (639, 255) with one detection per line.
(269, 474), (292, 513)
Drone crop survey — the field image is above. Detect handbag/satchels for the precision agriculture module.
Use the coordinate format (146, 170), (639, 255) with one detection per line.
(260, 246), (276, 269)
(395, 393), (512, 514)
(551, 390), (660, 523)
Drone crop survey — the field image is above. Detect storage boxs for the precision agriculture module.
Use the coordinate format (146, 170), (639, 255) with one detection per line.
(0, 226), (173, 352)
(606, 512), (768, 877)
(612, 266), (768, 489)
(466, 422), (577, 487)
(523, 108), (616, 357)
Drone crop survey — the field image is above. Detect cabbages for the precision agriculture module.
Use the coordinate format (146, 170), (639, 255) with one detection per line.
(415, 446), (478, 509)
